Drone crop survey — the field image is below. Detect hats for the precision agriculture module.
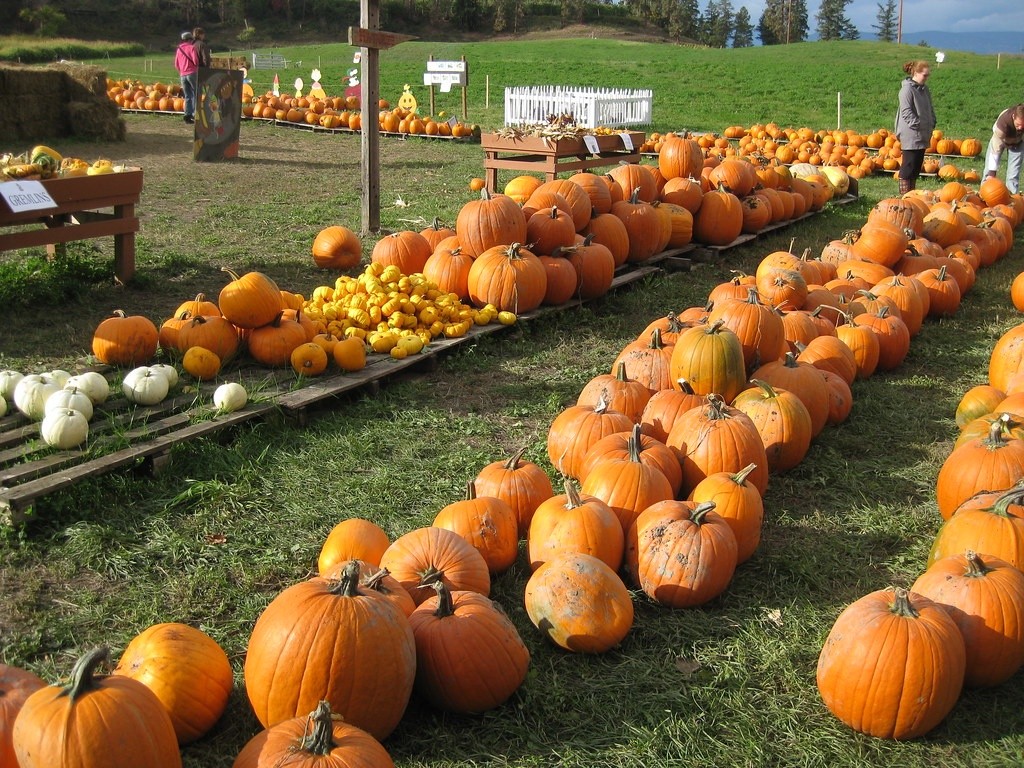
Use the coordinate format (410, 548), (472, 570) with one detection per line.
(180, 32), (194, 40)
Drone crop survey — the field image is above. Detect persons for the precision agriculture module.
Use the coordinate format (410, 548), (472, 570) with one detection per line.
(175, 27), (210, 124)
(894, 61), (937, 194)
(981, 103), (1024, 194)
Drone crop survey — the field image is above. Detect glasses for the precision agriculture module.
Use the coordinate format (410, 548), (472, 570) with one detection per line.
(921, 73), (930, 78)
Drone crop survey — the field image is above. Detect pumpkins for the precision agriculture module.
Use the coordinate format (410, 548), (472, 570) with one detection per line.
(0, 78), (1024, 768)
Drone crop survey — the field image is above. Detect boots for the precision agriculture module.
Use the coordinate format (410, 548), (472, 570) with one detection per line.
(898, 178), (917, 196)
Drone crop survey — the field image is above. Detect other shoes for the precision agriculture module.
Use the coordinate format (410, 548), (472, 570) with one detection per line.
(183, 115), (194, 124)
